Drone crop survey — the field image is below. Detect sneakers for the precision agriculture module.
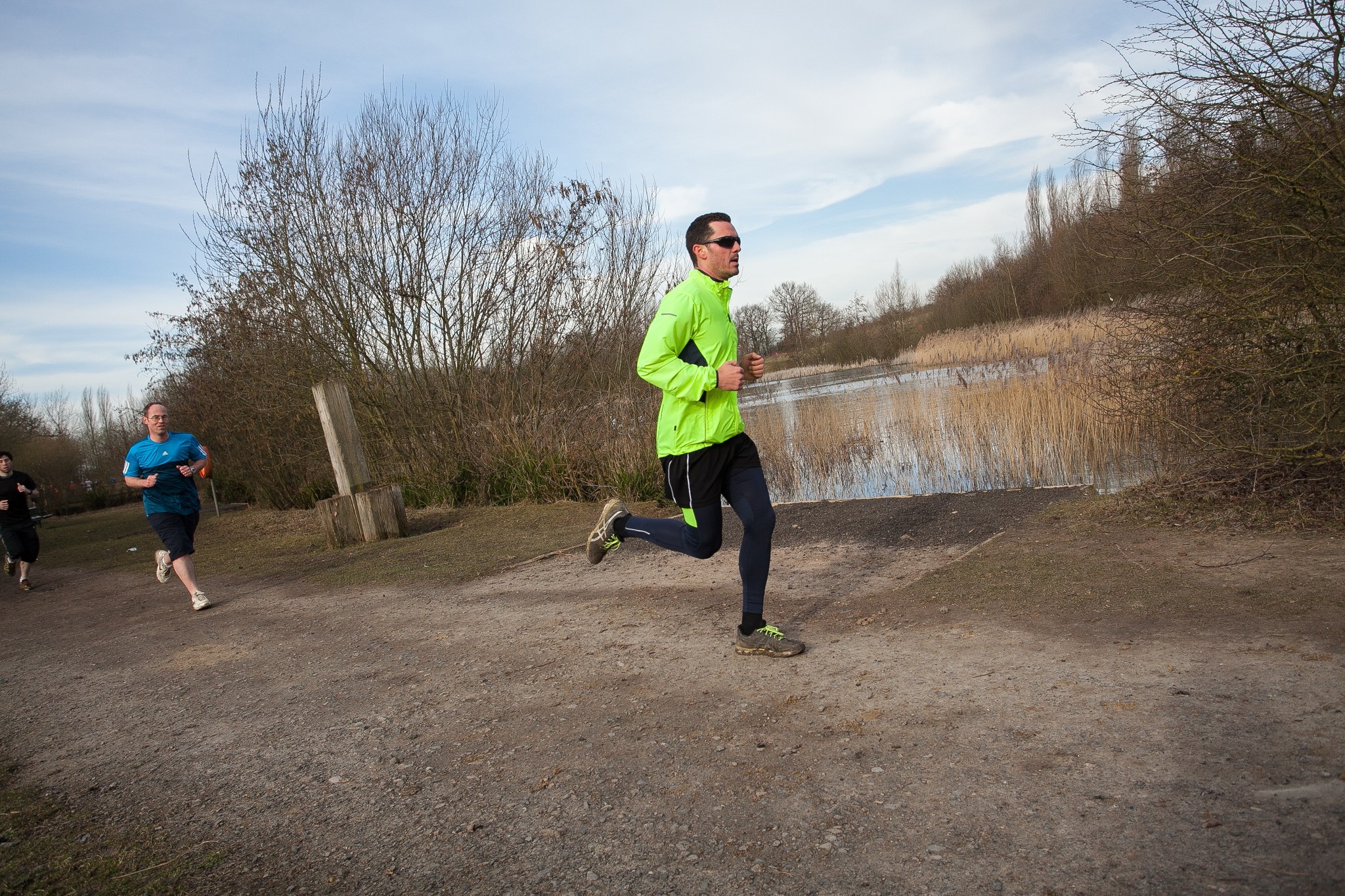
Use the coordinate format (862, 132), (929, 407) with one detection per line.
(4, 553), (16, 577)
(154, 549), (173, 584)
(19, 579), (31, 590)
(586, 499), (632, 564)
(735, 624), (806, 657)
(192, 591), (211, 610)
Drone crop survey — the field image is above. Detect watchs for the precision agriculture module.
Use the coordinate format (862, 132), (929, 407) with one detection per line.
(27, 489), (33, 495)
(189, 466), (196, 475)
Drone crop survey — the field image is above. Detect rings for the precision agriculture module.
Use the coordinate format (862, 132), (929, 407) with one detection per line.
(185, 472), (187, 474)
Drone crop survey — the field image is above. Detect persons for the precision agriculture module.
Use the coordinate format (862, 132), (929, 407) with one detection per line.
(586, 213), (805, 658)
(123, 402), (211, 611)
(0, 452), (42, 591)
(30, 476), (116, 507)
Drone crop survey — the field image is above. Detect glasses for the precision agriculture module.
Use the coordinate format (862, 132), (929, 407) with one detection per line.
(147, 415), (169, 421)
(699, 236), (741, 248)
